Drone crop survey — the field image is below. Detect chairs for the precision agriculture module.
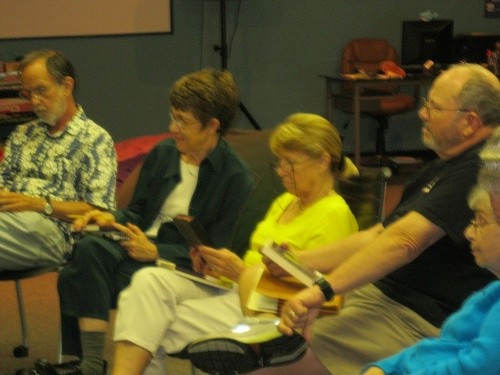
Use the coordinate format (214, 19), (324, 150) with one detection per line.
(338, 37), (421, 173)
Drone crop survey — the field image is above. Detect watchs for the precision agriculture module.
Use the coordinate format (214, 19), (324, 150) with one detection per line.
(44, 195), (53, 216)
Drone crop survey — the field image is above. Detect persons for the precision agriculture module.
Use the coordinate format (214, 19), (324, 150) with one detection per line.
(15, 67), (258, 375)
(111, 112), (361, 375)
(261, 62), (500, 375)
(360, 124), (500, 375)
(0, 49), (118, 271)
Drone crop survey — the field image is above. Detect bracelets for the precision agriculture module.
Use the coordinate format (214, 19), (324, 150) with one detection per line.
(313, 276), (335, 302)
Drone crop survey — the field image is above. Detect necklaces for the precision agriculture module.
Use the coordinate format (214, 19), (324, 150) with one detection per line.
(421, 175), (439, 193)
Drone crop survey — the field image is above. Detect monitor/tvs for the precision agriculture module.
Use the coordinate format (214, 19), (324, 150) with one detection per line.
(400, 21), (454, 72)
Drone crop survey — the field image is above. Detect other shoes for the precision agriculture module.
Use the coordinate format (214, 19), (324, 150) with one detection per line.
(35, 358), (108, 375)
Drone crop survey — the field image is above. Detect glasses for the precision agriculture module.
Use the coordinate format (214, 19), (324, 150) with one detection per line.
(168, 107), (201, 128)
(470, 211), (500, 231)
(19, 82), (52, 99)
(269, 149), (318, 171)
(417, 94), (478, 115)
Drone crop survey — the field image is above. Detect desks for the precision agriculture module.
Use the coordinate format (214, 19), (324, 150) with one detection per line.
(320, 72), (435, 170)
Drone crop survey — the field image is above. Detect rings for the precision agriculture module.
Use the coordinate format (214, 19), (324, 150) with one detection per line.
(288, 311), (296, 318)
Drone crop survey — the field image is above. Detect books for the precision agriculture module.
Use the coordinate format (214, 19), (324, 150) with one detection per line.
(263, 241), (320, 288)
(155, 258), (234, 291)
(174, 214), (218, 264)
(71, 223), (130, 241)
(247, 267), (342, 315)
